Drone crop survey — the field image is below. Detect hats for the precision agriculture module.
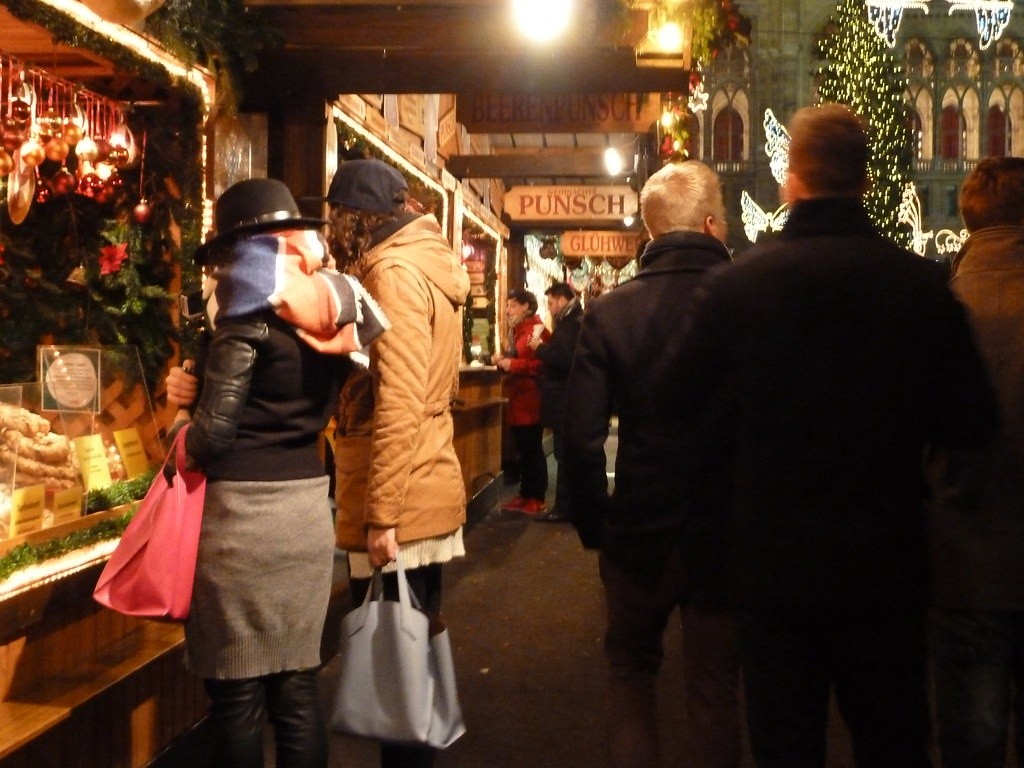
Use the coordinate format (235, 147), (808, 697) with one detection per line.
(301, 158), (408, 213)
(194, 177), (328, 264)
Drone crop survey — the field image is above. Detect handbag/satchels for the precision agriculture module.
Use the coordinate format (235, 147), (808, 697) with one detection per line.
(329, 549), (467, 750)
(93, 423), (208, 624)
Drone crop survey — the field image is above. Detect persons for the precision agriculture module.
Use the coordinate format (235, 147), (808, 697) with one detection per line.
(167, 177), (390, 768)
(490, 284), (584, 522)
(565, 105), (1023, 768)
(325, 158), (468, 767)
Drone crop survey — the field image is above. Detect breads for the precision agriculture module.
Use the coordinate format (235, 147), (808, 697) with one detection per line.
(0, 402), (77, 490)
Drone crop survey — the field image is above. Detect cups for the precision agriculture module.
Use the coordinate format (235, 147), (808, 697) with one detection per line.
(470, 345), (483, 363)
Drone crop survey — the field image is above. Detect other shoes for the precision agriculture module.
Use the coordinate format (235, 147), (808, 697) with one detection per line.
(521, 500), (546, 514)
(537, 511), (568, 522)
(502, 495), (524, 512)
